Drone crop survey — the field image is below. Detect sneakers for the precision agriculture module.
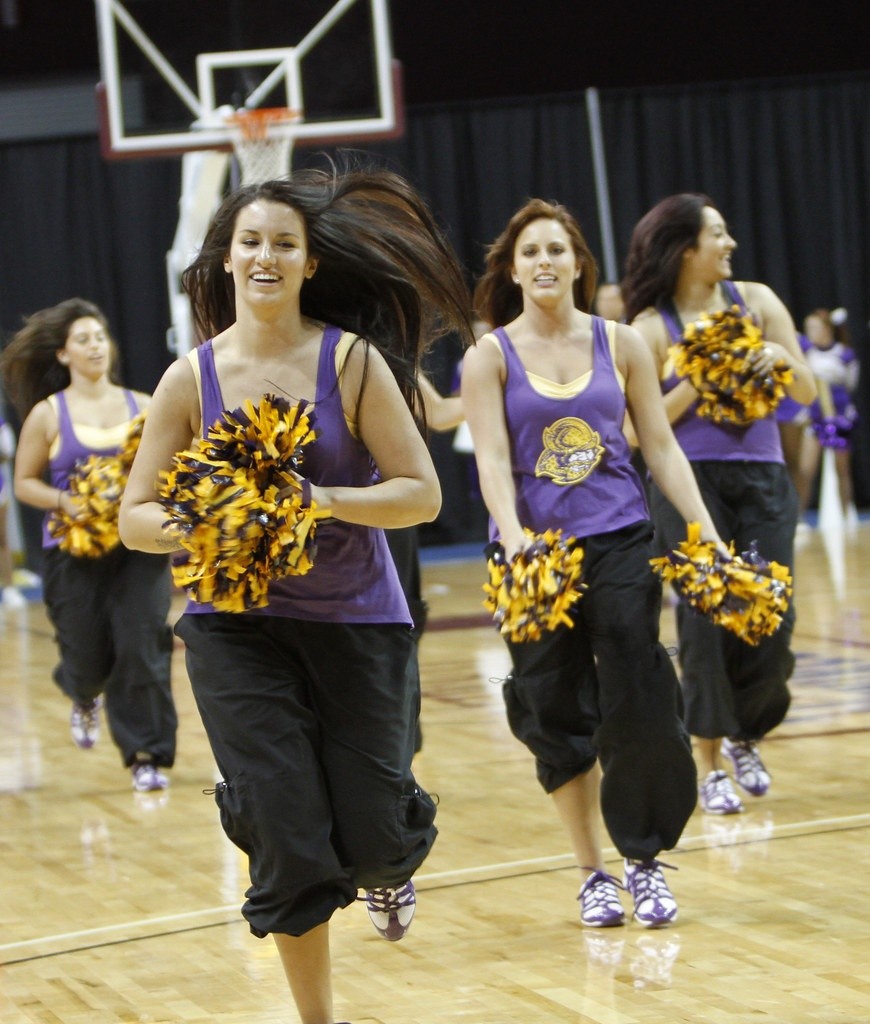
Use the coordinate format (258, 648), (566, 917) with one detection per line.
(621, 853), (681, 926)
(71, 697), (101, 747)
(131, 762), (168, 791)
(721, 736), (772, 796)
(574, 865), (626, 926)
(354, 879), (416, 941)
(697, 770), (742, 815)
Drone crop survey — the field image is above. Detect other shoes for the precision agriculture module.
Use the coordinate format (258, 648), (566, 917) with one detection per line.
(794, 523), (813, 551)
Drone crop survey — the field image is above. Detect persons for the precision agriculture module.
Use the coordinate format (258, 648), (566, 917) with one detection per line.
(622, 192), (817, 813)
(460, 199), (741, 929)
(118, 162), (479, 1024)
(0, 284), (860, 793)
